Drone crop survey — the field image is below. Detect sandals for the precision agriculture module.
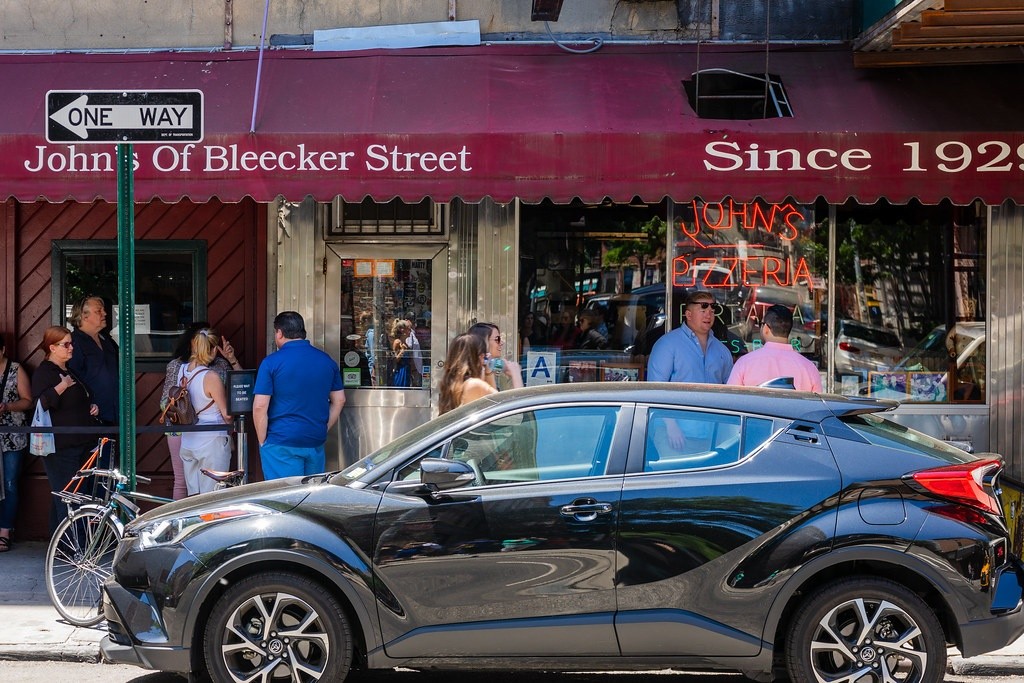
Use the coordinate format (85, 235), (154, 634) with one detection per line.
(0, 537), (12, 551)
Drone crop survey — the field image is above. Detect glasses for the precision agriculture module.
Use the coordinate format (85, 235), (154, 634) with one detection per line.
(759, 322), (771, 329)
(56, 342), (73, 348)
(579, 319), (586, 324)
(691, 301), (718, 309)
(489, 336), (500, 344)
(562, 316), (570, 321)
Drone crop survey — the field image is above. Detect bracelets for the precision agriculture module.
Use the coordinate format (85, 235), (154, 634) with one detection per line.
(232, 360), (238, 365)
(485, 370), (493, 376)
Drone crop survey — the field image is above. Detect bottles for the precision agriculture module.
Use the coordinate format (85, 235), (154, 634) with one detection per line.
(483, 358), (507, 371)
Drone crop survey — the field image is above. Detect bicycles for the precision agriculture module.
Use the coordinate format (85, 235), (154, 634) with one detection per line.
(45, 436), (246, 628)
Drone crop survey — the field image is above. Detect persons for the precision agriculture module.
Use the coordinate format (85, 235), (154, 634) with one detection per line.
(392, 318), (423, 386)
(359, 310), (376, 386)
(394, 537), (545, 558)
(176, 328), (235, 496)
(520, 306), (608, 349)
(0, 333), (32, 551)
(647, 291), (734, 459)
(252, 311), (347, 481)
(439, 323), (524, 470)
(160, 321), (244, 498)
(32, 326), (100, 561)
(726, 309), (822, 392)
(66, 296), (120, 498)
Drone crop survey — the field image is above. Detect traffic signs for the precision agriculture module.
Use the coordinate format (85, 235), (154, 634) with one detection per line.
(45, 87), (204, 143)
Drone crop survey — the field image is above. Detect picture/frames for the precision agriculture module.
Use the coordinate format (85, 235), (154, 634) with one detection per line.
(601, 363), (645, 382)
(867, 371), (950, 402)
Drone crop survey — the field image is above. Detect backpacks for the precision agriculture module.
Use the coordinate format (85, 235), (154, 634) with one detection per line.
(159, 368), (215, 426)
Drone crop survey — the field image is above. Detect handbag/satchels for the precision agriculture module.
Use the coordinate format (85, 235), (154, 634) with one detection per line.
(412, 371), (422, 387)
(95, 419), (113, 426)
(30, 398), (56, 457)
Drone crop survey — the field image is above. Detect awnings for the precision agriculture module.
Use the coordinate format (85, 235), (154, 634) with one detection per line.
(0, 45), (1024, 205)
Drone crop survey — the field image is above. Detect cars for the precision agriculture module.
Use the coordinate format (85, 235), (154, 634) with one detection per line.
(99, 380), (1024, 683)
(520, 243), (988, 406)
